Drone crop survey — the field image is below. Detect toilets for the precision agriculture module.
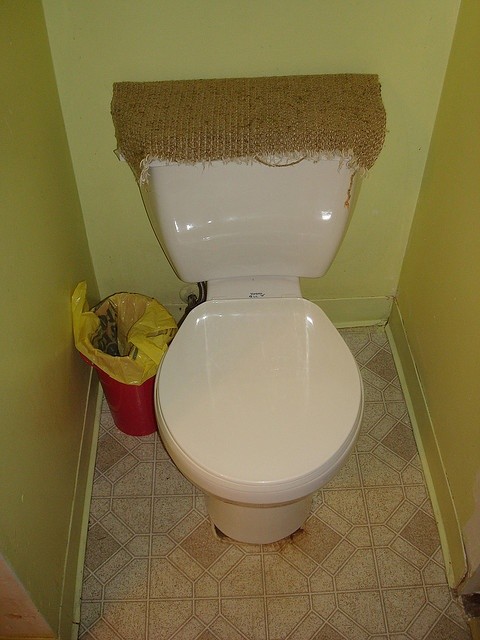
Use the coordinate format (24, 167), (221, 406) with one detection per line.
(113, 80), (374, 545)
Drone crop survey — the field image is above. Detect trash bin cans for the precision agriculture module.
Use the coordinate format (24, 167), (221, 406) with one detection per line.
(81, 293), (174, 440)
